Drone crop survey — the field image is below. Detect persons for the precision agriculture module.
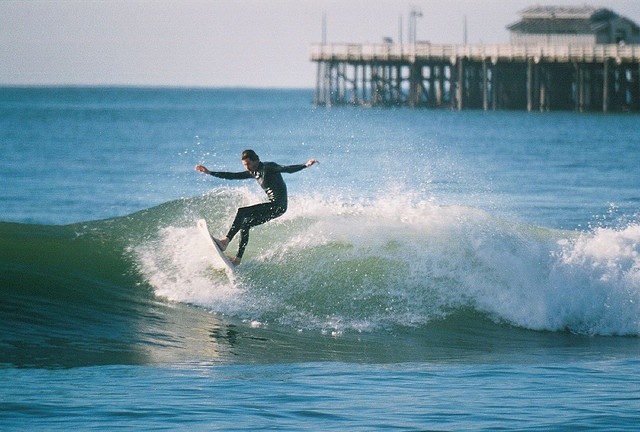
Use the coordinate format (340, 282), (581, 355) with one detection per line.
(195, 150), (319, 267)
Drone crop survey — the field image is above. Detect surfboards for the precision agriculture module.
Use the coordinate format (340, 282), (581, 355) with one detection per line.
(197, 219), (236, 281)
